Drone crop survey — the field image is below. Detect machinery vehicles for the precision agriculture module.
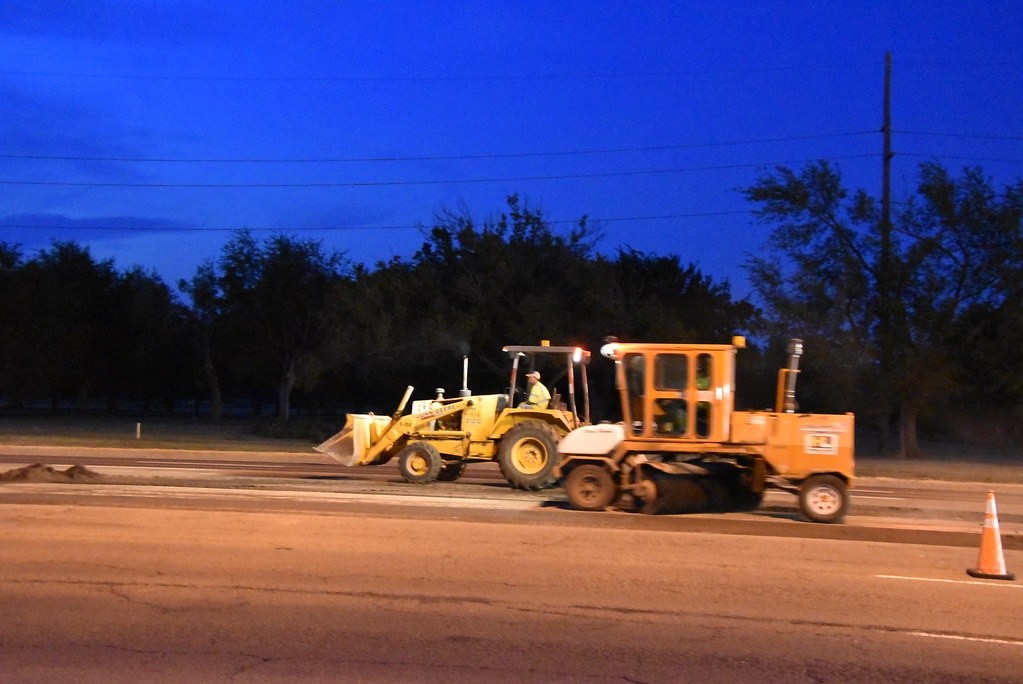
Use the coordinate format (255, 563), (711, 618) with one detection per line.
(563, 336), (857, 525)
(313, 343), (594, 491)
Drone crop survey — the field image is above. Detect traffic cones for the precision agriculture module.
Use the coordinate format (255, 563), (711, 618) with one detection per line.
(965, 488), (1015, 580)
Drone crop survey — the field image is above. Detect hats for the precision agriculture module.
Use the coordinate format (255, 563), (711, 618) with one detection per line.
(525, 371), (540, 379)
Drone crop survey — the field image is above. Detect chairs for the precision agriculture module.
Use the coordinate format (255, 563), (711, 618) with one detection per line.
(548, 388), (561, 409)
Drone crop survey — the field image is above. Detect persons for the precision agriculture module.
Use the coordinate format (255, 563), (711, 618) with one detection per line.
(664, 365), (709, 433)
(518, 371), (551, 409)
